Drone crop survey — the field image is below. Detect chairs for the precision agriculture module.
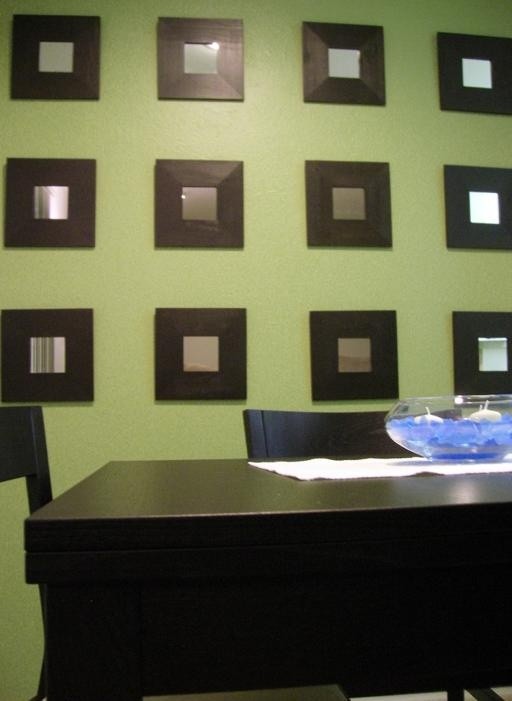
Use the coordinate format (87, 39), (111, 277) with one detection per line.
(239, 404), (467, 462)
(0, 404), (348, 700)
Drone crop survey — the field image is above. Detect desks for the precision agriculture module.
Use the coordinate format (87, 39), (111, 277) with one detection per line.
(22, 448), (511, 701)
